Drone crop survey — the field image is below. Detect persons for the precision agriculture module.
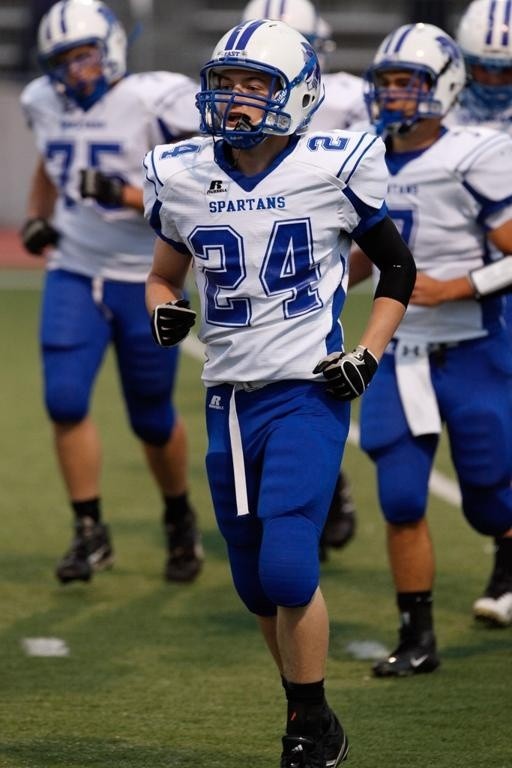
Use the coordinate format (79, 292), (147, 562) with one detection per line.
(18, 0), (213, 582)
(240, 1), (356, 562)
(443, 1), (511, 632)
(347, 21), (511, 680)
(141, 20), (417, 766)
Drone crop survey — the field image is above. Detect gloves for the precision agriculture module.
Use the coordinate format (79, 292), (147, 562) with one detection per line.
(78, 166), (130, 213)
(311, 342), (380, 404)
(17, 214), (64, 258)
(147, 296), (198, 350)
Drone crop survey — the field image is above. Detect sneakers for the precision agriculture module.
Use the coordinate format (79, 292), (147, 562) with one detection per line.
(320, 464), (360, 552)
(159, 507), (208, 589)
(278, 707), (352, 768)
(470, 549), (512, 630)
(370, 625), (441, 680)
(54, 521), (116, 587)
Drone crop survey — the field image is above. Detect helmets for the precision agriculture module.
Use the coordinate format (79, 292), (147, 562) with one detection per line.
(370, 21), (469, 120)
(33, 0), (132, 97)
(240, 1), (335, 77)
(453, 0), (512, 68)
(203, 16), (326, 139)
